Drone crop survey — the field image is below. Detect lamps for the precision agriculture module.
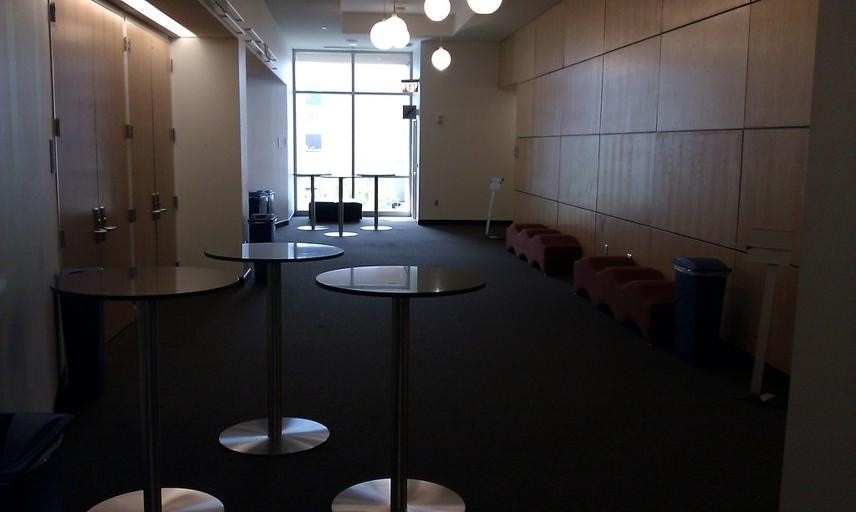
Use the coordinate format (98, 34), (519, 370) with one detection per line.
(370, 0), (409, 50)
(467, 0), (503, 16)
(431, 46), (452, 71)
(423, 0), (451, 23)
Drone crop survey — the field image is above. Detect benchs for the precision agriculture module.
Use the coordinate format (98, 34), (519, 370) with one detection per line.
(309, 201), (362, 222)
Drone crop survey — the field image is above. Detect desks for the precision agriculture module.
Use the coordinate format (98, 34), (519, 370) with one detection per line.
(293, 172), (333, 231)
(321, 175), (361, 237)
(49, 266), (243, 512)
(358, 173), (396, 231)
(314, 264), (487, 512)
(203, 242), (343, 456)
